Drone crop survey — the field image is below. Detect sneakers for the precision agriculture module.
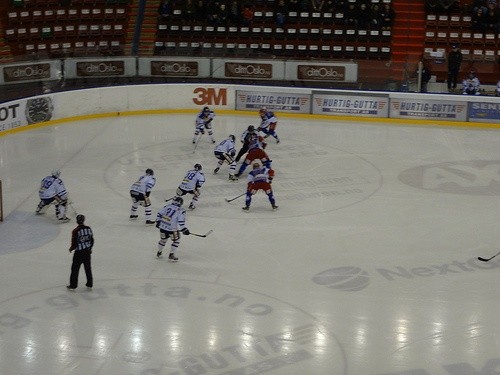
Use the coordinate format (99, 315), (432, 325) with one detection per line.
(276, 137), (280, 144)
(130, 215), (138, 220)
(35, 211), (46, 215)
(60, 217), (71, 223)
(188, 205), (196, 210)
(231, 176), (238, 182)
(85, 283), (93, 289)
(214, 168), (216, 175)
(272, 206), (280, 211)
(157, 251), (162, 256)
(67, 286), (76, 291)
(241, 207), (249, 212)
(234, 174), (239, 178)
(263, 135), (269, 139)
(146, 220), (155, 226)
(168, 253), (179, 262)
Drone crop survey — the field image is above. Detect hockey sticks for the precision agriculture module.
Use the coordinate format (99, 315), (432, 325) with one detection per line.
(178, 229), (213, 238)
(477, 251), (500, 262)
(165, 196), (173, 202)
(224, 192), (246, 202)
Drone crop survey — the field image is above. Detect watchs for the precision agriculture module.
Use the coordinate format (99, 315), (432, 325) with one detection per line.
(26, 97), (53, 124)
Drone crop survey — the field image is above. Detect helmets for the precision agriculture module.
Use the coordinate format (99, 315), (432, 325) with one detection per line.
(51, 168), (61, 177)
(173, 197), (183, 205)
(253, 163), (260, 169)
(202, 107), (209, 112)
(247, 125), (254, 133)
(194, 164), (202, 171)
(228, 135), (236, 142)
(145, 168), (154, 176)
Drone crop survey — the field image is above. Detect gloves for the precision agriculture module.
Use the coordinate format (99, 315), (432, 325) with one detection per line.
(156, 222), (160, 228)
(183, 230), (190, 235)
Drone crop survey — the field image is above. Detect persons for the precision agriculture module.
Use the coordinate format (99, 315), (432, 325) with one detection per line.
(446, 45), (462, 89)
(473, 0), (500, 32)
(158, 0), (395, 31)
(414, 61), (430, 92)
(426, 0), (473, 12)
(256, 108), (279, 143)
(234, 125), (272, 177)
(214, 135), (238, 181)
(242, 163), (278, 210)
(156, 197), (190, 261)
(35, 168), (69, 222)
(463, 71), (480, 95)
(66, 215), (94, 289)
(192, 107), (216, 144)
(176, 164), (206, 209)
(130, 169), (156, 224)
